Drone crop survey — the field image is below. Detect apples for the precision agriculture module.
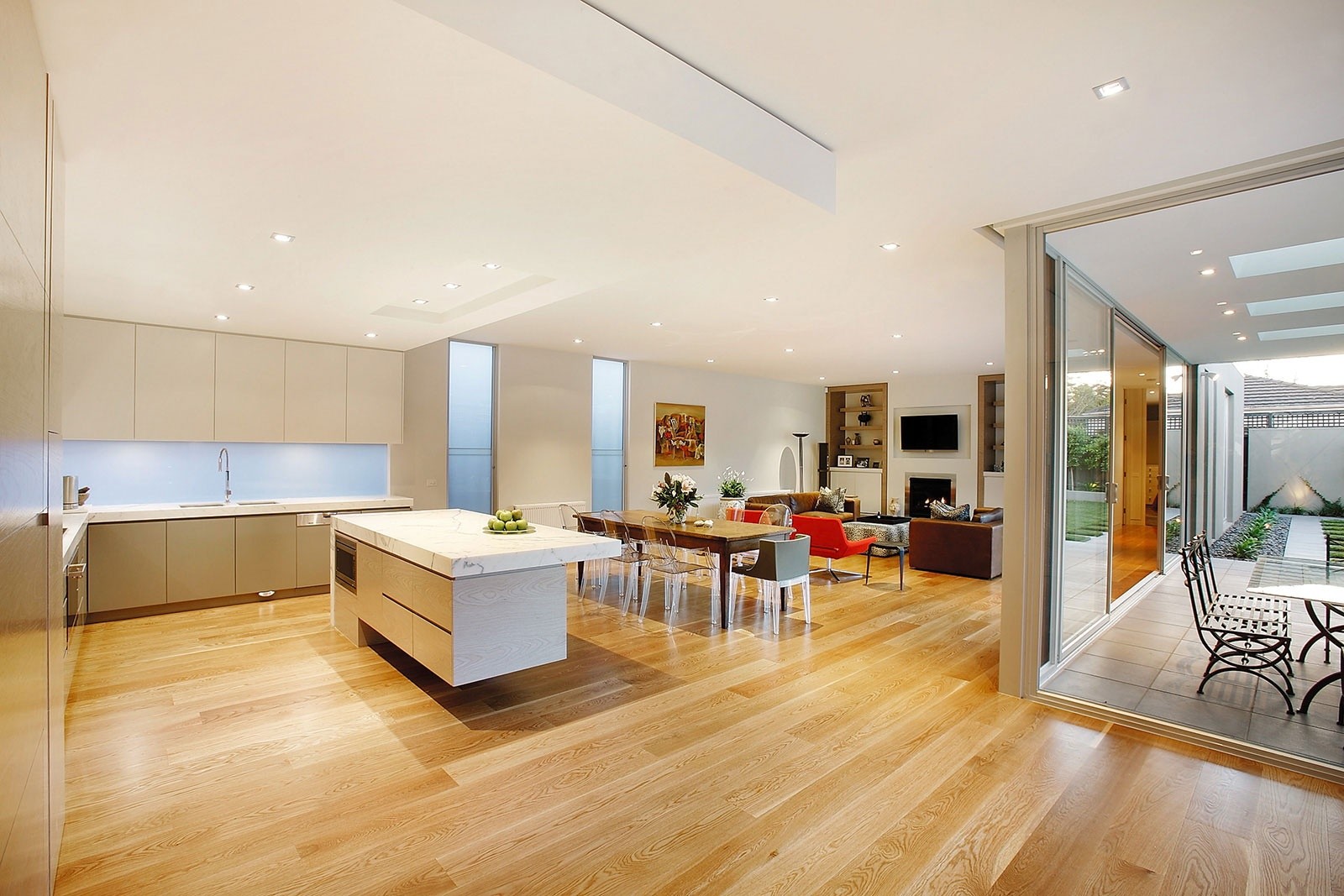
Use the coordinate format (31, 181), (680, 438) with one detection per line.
(496, 510), (505, 519)
(512, 510), (523, 520)
(493, 520), (504, 530)
(488, 518), (498, 529)
(516, 519), (527, 530)
(505, 521), (517, 531)
(500, 510), (512, 522)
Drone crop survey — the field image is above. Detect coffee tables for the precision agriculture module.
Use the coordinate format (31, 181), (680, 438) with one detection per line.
(842, 514), (912, 558)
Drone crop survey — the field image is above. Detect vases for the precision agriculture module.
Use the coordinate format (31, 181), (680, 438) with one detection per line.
(668, 507), (687, 523)
(889, 496), (902, 516)
(716, 498), (747, 521)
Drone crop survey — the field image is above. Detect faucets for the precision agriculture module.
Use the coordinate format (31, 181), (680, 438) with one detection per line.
(218, 448), (232, 502)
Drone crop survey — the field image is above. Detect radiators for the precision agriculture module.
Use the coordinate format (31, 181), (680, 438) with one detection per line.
(512, 500), (587, 532)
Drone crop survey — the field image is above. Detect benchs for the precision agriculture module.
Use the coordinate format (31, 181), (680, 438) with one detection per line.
(1323, 532), (1344, 665)
(1179, 525), (1296, 717)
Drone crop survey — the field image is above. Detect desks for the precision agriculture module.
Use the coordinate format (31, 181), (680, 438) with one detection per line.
(1244, 551), (1344, 728)
(576, 502), (799, 634)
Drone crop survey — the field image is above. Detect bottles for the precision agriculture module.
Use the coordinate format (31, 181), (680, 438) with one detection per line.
(855, 433), (861, 445)
(889, 497), (900, 515)
(846, 436), (851, 445)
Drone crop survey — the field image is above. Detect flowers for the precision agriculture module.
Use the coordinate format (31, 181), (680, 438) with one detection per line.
(648, 471), (709, 516)
(714, 465), (754, 498)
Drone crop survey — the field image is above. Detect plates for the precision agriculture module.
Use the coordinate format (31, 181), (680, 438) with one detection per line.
(483, 526), (535, 532)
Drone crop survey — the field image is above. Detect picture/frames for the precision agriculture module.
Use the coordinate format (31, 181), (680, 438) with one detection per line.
(652, 401), (707, 471)
(836, 454), (854, 468)
(855, 457), (870, 469)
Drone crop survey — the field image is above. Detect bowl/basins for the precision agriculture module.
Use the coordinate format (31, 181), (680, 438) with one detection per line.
(78, 493), (89, 506)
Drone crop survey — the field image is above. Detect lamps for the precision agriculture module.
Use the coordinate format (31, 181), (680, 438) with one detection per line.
(790, 431), (810, 494)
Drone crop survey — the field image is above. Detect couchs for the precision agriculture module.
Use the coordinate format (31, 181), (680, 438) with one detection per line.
(745, 491), (861, 522)
(910, 504), (1005, 580)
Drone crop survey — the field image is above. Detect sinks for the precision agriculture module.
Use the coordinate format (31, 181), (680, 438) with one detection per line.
(237, 501), (281, 505)
(179, 504), (225, 508)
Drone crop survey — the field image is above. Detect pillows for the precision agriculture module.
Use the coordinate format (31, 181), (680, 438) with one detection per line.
(928, 499), (974, 522)
(813, 486), (847, 514)
(786, 493), (804, 515)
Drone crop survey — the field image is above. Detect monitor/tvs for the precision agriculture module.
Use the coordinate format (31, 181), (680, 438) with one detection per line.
(900, 414), (959, 451)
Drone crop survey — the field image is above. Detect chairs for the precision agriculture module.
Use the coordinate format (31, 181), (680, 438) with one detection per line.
(637, 513), (721, 633)
(725, 507), (766, 524)
(716, 505), (747, 523)
(758, 503), (793, 528)
(789, 514), (878, 584)
(558, 502), (605, 603)
(597, 508), (667, 617)
(728, 534), (812, 635)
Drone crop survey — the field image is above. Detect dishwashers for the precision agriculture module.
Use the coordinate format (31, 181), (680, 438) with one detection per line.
(297, 511), (361, 589)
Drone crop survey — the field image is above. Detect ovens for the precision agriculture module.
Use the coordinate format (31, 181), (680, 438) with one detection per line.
(335, 531), (357, 596)
(66, 537), (87, 655)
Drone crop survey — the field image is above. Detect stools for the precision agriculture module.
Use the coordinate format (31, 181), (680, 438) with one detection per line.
(864, 540), (911, 591)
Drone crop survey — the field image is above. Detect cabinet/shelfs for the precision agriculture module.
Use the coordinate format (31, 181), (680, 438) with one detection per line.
(0, 0), (62, 896)
(976, 372), (1008, 508)
(826, 381), (888, 514)
(331, 528), (569, 688)
(60, 312), (407, 447)
(1146, 463), (1159, 507)
(53, 508), (414, 715)
